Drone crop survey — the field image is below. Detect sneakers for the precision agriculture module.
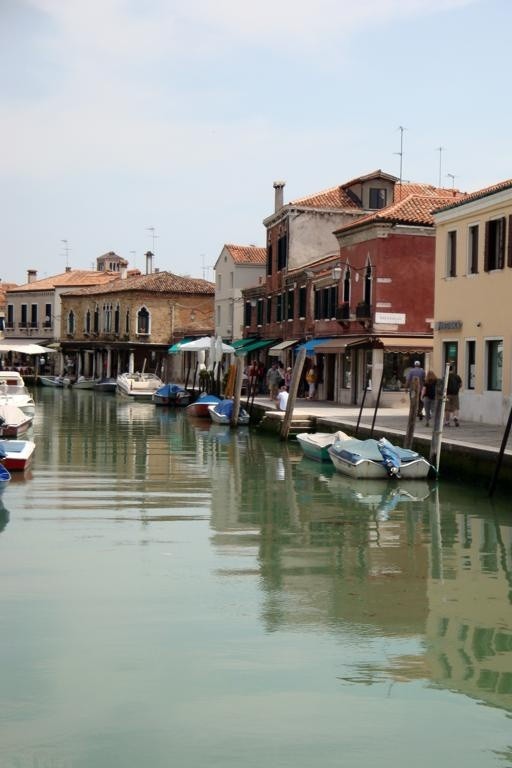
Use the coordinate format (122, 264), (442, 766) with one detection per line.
(424, 416), (460, 427)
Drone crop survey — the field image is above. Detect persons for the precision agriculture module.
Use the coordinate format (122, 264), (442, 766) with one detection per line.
(247, 357), (317, 411)
(404, 361), (426, 417)
(420, 371), (438, 427)
(445, 366), (462, 427)
(40, 355), (46, 376)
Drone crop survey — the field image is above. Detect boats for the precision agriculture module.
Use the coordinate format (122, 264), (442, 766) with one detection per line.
(152, 385), (191, 405)
(0, 370), (35, 469)
(296, 431), (432, 479)
(184, 395), (222, 417)
(39, 377), (117, 391)
(208, 400), (250, 424)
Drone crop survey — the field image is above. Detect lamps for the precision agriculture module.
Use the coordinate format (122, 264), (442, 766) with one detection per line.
(189, 307), (214, 322)
(248, 293), (279, 311)
(330, 261), (376, 281)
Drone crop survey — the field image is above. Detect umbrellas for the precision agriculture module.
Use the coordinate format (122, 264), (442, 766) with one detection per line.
(213, 336), (224, 384)
(180, 337), (235, 353)
(207, 335), (214, 380)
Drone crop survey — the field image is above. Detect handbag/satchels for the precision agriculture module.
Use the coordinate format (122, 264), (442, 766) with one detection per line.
(305, 374), (318, 383)
